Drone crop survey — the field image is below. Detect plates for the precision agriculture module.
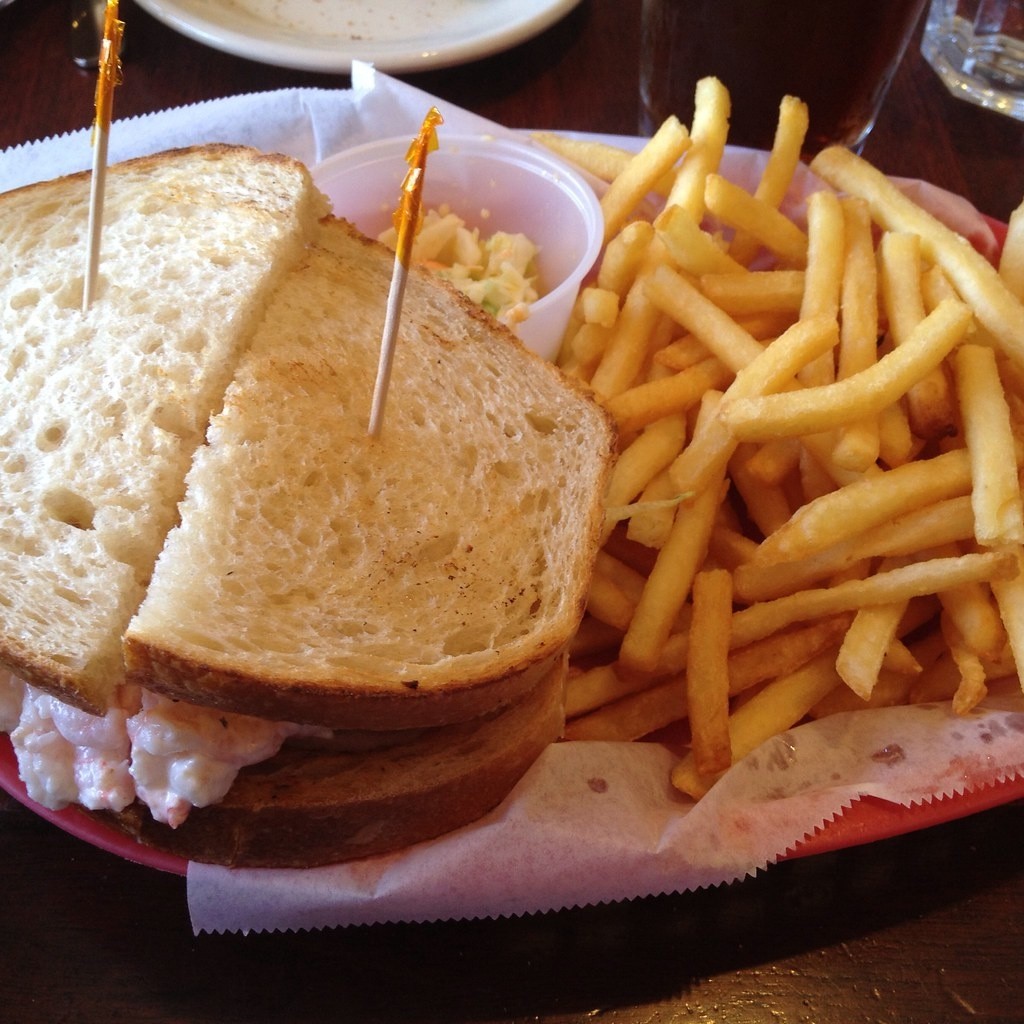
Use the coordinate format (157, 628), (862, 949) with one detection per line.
(1, 214), (1023, 878)
(134, 0), (586, 75)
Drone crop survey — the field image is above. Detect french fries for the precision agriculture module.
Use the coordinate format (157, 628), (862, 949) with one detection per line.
(521, 74), (1024, 794)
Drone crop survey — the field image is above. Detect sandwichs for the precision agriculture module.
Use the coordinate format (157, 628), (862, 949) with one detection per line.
(1, 146), (607, 867)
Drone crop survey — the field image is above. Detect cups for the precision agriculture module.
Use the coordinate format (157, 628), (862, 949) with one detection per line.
(920, 0), (1024, 121)
(636, 1), (926, 165)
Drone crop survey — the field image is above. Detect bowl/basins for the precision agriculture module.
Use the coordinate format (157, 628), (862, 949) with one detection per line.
(307, 136), (603, 367)
